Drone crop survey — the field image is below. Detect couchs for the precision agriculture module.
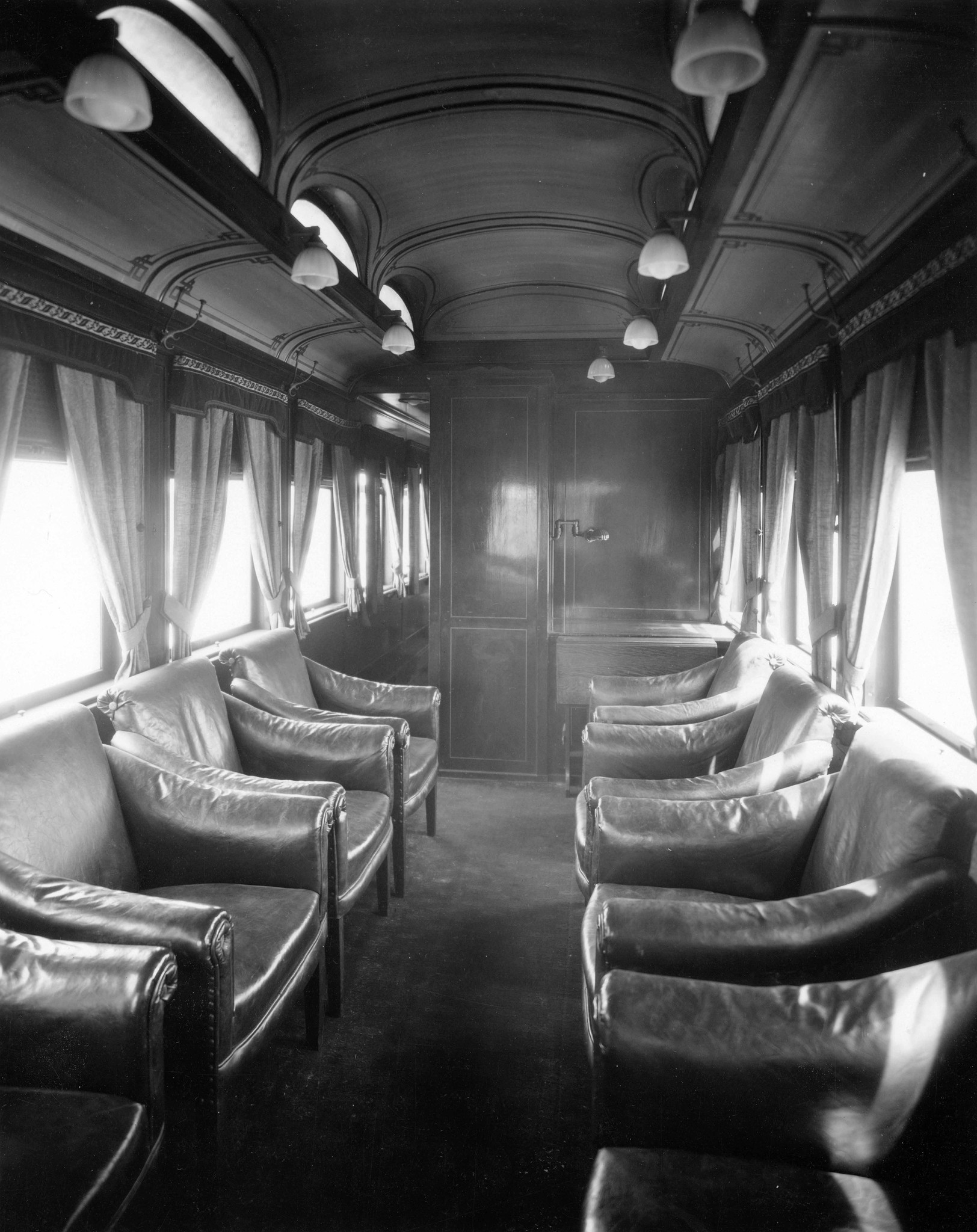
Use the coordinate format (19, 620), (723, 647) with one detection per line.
(587, 632), (783, 723)
(572, 954), (977, 1230)
(581, 719), (977, 1064)
(0, 698), (335, 1173)
(0, 927), (179, 1232)
(220, 626), (443, 898)
(98, 653), (396, 1021)
(573, 663), (844, 900)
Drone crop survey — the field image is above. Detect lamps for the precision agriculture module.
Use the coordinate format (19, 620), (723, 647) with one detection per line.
(63, 15), (151, 134)
(584, 2), (768, 384)
(378, 308), (416, 355)
(290, 223), (341, 293)
